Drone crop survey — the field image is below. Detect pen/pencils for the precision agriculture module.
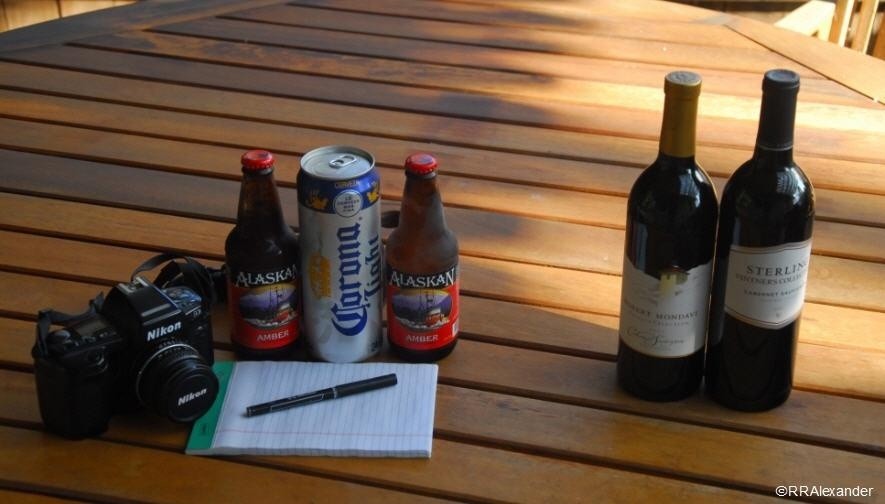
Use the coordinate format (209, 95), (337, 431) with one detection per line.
(247, 374), (398, 417)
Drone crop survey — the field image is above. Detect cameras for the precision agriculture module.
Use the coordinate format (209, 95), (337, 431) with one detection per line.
(32, 273), (220, 440)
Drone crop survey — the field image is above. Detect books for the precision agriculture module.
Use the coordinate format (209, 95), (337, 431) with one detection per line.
(184, 357), (438, 458)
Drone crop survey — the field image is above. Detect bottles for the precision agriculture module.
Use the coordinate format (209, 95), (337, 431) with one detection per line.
(224, 149), (304, 359)
(383, 152), (460, 366)
(616, 68), (816, 416)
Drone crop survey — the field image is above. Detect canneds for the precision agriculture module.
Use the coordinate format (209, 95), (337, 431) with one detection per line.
(296, 144), (384, 363)
